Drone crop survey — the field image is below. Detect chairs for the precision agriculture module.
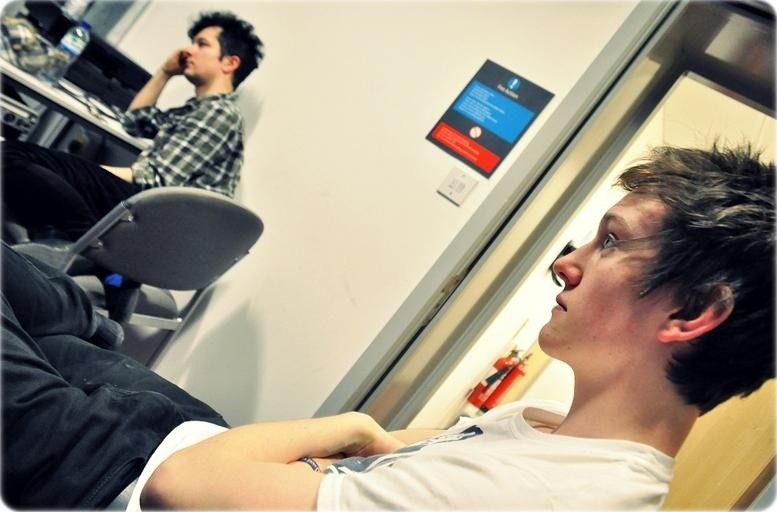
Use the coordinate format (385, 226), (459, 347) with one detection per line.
(4, 186), (264, 371)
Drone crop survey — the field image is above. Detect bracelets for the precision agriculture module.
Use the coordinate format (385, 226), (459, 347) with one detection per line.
(297, 455), (321, 473)
(161, 67), (175, 77)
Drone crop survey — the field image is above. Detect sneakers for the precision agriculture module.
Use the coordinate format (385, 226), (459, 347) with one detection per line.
(91, 307), (125, 349)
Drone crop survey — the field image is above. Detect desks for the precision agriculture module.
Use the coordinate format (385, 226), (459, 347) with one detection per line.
(0, 57), (158, 156)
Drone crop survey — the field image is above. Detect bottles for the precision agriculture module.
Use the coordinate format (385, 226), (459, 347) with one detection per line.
(36, 19), (92, 88)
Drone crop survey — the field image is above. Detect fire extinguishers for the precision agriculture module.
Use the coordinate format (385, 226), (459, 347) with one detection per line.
(467, 347), (527, 414)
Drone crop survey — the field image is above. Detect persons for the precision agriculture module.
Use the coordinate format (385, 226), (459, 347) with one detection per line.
(1, 139), (777, 512)
(2, 9), (266, 328)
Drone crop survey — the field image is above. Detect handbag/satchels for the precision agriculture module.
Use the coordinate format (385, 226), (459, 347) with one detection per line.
(0, 16), (69, 73)
